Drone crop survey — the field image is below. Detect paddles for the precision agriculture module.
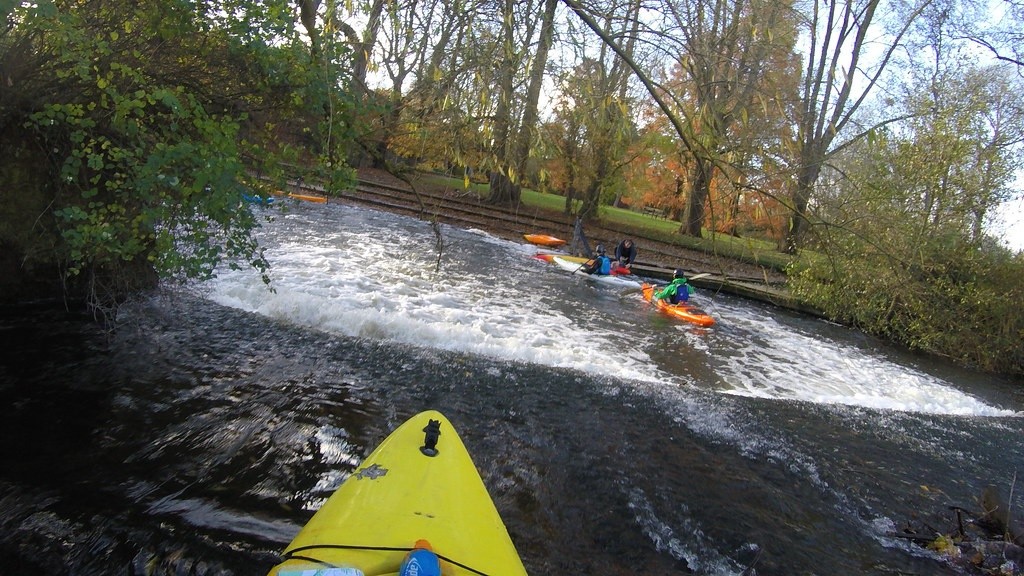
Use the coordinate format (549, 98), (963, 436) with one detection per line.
(570, 253), (596, 276)
(615, 270), (712, 300)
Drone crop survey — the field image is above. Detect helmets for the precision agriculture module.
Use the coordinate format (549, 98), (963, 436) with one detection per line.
(673, 269), (684, 276)
(596, 245), (605, 252)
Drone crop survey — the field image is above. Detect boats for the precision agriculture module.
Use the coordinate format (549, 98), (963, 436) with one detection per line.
(642, 283), (715, 327)
(525, 233), (566, 246)
(535, 252), (647, 290)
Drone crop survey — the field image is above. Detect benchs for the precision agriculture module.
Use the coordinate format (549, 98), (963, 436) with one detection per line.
(642, 201), (672, 221)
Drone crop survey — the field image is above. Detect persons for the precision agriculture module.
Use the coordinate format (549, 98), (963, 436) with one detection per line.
(614, 239), (638, 268)
(582, 244), (611, 275)
(651, 268), (695, 305)
